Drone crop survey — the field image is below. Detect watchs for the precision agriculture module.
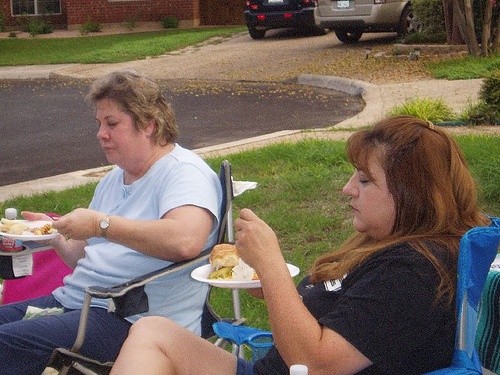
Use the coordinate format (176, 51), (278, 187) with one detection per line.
(99, 213), (111, 239)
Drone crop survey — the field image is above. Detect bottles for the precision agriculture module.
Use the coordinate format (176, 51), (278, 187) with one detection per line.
(290, 364), (308, 375)
(0, 208), (26, 280)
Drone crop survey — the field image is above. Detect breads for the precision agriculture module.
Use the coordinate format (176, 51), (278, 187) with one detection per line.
(209, 244), (258, 279)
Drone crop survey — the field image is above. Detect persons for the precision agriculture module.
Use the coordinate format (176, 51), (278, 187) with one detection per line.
(0, 71), (223, 375)
(111, 115), (492, 375)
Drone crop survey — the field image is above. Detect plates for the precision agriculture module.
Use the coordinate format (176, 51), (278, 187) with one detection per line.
(0, 220), (58, 240)
(191, 258), (300, 288)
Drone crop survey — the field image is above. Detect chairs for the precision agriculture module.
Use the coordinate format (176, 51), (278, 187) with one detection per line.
(213, 216), (500, 375)
(41, 160), (247, 375)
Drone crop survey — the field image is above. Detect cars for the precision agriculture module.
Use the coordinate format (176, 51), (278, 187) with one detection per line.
(243, 0), (330, 39)
(314, 0), (423, 44)
